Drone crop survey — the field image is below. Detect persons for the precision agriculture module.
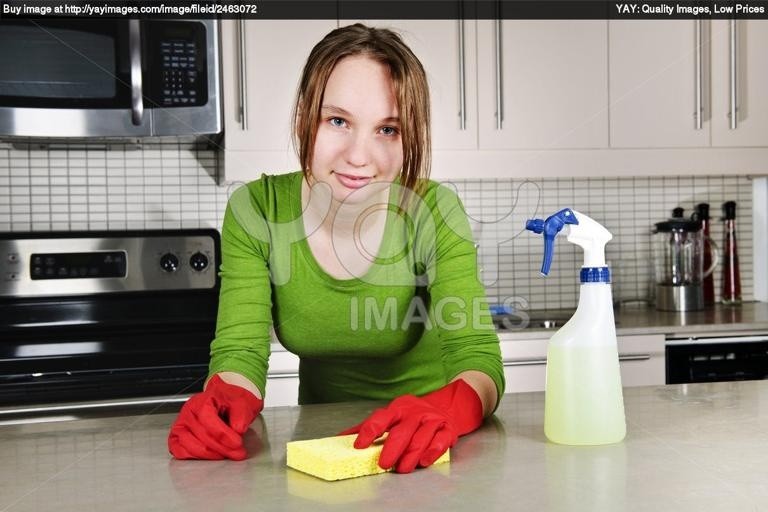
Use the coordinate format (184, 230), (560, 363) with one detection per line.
(167, 23), (507, 475)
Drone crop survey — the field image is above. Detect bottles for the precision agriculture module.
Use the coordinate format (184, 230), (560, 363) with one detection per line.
(669, 201), (743, 305)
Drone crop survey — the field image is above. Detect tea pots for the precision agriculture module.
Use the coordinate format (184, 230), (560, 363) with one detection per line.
(650, 218), (720, 313)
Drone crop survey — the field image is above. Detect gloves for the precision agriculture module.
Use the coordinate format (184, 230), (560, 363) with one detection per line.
(336, 378), (485, 476)
(165, 373), (266, 462)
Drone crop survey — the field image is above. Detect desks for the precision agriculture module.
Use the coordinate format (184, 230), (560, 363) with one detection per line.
(0, 380), (768, 512)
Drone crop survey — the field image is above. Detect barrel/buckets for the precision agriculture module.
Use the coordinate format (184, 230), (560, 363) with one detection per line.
(527, 211), (627, 445)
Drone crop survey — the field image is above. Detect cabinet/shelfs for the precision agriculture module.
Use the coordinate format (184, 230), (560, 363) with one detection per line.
(257, 335), (667, 405)
(215, 19), (768, 188)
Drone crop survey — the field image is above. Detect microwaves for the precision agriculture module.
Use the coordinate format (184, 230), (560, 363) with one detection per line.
(0, 17), (222, 151)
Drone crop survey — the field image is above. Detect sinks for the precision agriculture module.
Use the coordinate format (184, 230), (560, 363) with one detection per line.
(495, 317), (565, 332)
